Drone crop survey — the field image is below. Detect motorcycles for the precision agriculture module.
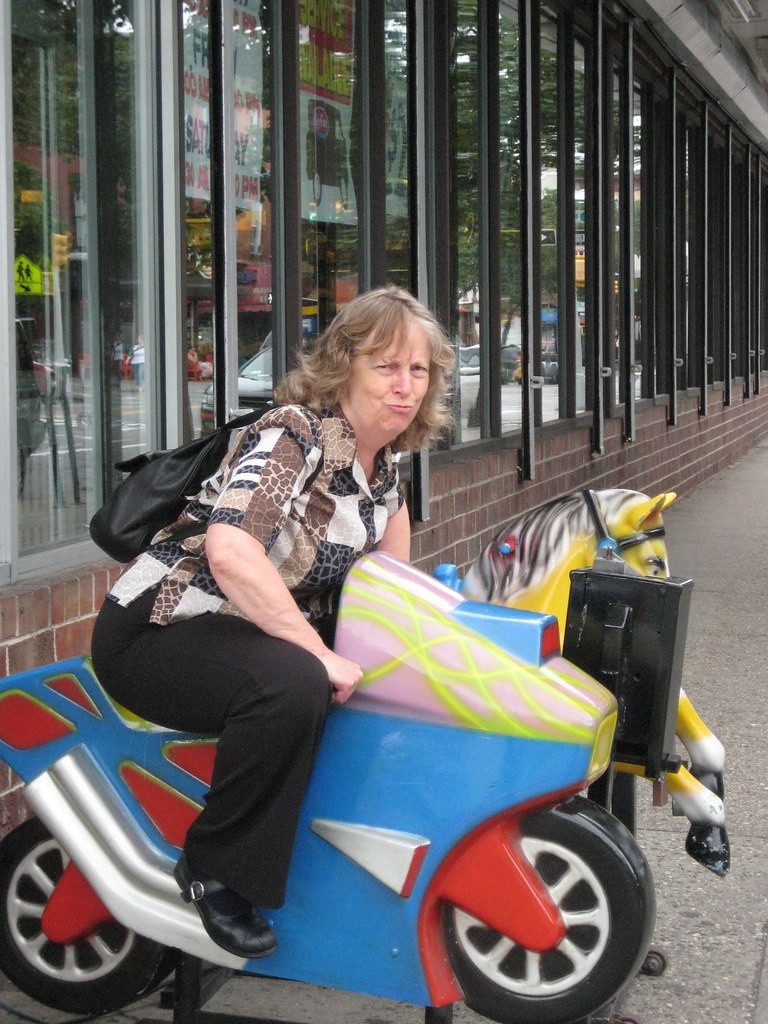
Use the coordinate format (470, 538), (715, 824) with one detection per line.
(0, 553), (657, 1024)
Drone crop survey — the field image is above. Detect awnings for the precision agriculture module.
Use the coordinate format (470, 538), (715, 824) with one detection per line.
(184, 270), (251, 345)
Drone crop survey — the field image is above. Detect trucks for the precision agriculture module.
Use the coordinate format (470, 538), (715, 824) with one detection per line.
(542, 312), (582, 383)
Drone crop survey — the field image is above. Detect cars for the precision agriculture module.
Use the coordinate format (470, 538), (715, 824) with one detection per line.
(501, 344), (523, 385)
(200, 345), (274, 435)
(460, 343), (482, 428)
(15, 320), (47, 498)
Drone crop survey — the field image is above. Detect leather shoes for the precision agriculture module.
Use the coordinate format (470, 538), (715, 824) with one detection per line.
(174, 853), (278, 958)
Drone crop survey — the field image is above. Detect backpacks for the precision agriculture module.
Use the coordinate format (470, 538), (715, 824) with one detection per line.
(89, 406), (325, 563)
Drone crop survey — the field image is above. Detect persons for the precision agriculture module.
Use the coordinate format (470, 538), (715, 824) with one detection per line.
(90, 283), (454, 956)
(205, 344), (214, 361)
(188, 346), (203, 382)
(110, 332), (145, 393)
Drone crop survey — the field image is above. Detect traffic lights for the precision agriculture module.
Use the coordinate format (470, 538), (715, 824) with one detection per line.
(52, 233), (68, 266)
(541, 229), (557, 246)
(615, 280), (619, 294)
